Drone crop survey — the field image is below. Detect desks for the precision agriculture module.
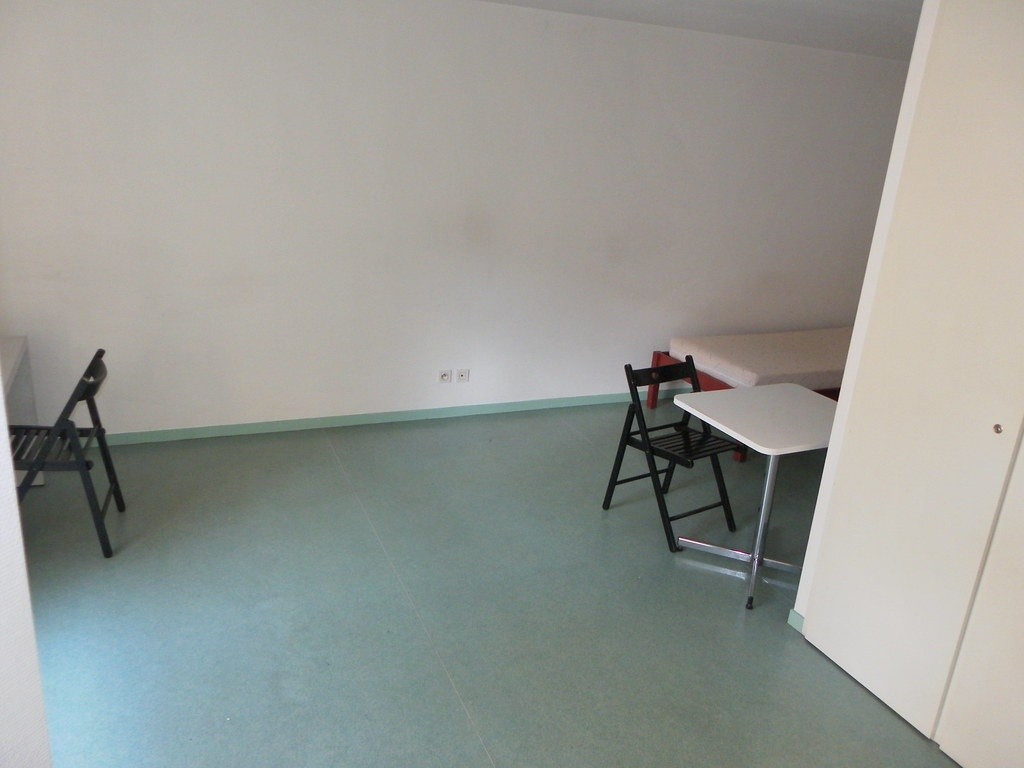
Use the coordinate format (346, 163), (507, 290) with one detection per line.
(0, 335), (45, 488)
(674, 383), (839, 610)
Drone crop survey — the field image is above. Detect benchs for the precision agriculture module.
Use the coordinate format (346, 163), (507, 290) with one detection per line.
(647, 325), (855, 462)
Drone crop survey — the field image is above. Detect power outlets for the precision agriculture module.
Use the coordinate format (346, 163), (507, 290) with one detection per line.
(438, 369), (452, 383)
(455, 368), (469, 382)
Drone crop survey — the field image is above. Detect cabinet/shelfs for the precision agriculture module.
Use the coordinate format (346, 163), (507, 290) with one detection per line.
(802, 0), (1024, 768)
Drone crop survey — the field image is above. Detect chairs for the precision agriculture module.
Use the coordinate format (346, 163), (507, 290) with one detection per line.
(6, 349), (126, 559)
(601, 354), (743, 553)
